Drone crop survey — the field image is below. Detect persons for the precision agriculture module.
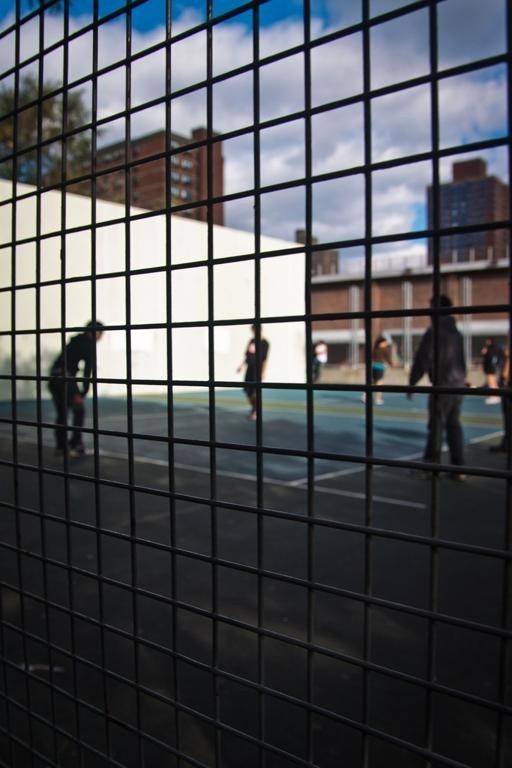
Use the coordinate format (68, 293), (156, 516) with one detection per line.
(408, 295), (467, 481)
(360, 338), (393, 404)
(311, 340), (328, 382)
(489, 381), (510, 452)
(49, 322), (103, 455)
(482, 336), (510, 404)
(237, 325), (268, 421)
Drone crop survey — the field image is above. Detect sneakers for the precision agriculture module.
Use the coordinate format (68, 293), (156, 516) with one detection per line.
(484, 395), (502, 406)
(489, 442), (505, 452)
(411, 463), (467, 480)
(360, 395), (383, 405)
(56, 440), (86, 456)
